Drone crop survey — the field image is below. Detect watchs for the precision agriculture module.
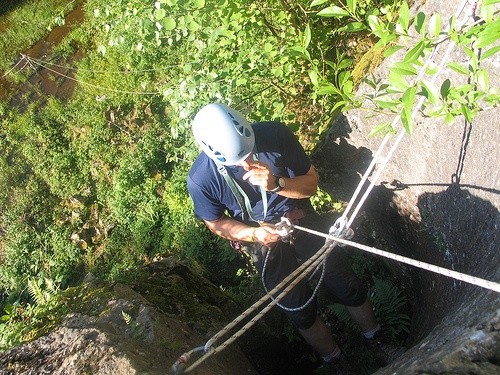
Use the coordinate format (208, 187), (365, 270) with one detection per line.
(269, 175), (286, 194)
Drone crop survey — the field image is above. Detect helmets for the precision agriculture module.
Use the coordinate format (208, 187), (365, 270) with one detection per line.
(192, 103), (255, 166)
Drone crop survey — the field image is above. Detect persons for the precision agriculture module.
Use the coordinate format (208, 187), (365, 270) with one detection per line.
(187, 103), (406, 375)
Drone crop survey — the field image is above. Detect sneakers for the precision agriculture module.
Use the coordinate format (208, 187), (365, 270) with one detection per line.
(326, 349), (367, 375)
(364, 330), (407, 365)
